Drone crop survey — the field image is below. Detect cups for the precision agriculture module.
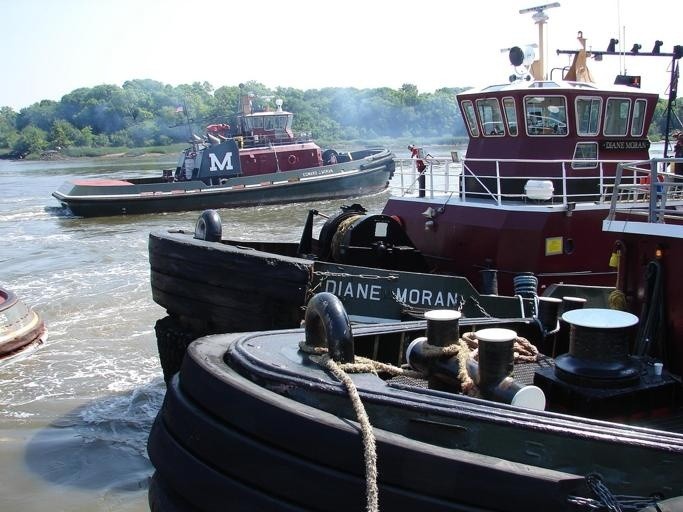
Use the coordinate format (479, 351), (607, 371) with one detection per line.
(654, 362), (663, 376)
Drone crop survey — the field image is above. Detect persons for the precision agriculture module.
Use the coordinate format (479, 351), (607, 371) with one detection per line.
(408, 145), (427, 197)
